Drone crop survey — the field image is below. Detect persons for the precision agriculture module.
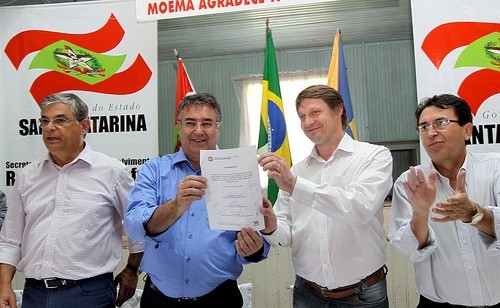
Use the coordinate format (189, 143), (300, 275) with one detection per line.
(261, 85), (393, 308)
(0, 94), (144, 308)
(124, 92), (270, 308)
(388, 94), (500, 308)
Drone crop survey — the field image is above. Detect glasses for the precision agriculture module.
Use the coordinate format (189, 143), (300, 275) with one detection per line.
(39, 118), (86, 128)
(416, 118), (459, 135)
(178, 120), (219, 130)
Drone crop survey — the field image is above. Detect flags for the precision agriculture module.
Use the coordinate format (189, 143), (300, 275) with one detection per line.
(327, 31), (358, 140)
(258, 28), (292, 205)
(172, 60), (194, 152)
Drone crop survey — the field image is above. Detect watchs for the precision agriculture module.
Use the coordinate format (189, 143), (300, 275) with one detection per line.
(462, 202), (484, 225)
(126, 264), (142, 275)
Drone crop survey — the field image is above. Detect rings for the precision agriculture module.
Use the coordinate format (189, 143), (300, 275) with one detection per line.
(277, 164), (280, 170)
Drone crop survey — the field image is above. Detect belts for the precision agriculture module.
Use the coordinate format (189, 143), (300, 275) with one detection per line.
(145, 275), (235, 305)
(296, 266), (386, 298)
(26, 273), (113, 288)
